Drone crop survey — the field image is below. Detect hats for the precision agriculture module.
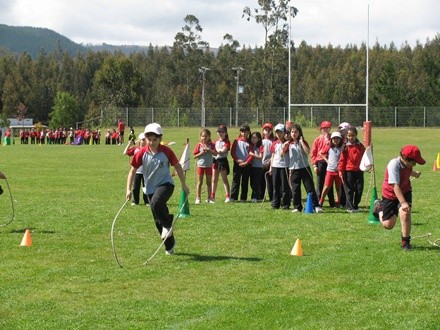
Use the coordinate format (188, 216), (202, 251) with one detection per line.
(401, 145), (426, 165)
(264, 123), (273, 129)
(275, 124), (286, 132)
(216, 125), (227, 132)
(144, 123), (162, 135)
(321, 121), (332, 128)
(139, 132), (145, 139)
(331, 132), (342, 139)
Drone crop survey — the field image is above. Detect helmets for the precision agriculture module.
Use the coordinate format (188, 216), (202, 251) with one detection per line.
(338, 123), (351, 132)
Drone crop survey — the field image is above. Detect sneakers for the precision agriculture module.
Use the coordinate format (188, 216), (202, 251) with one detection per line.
(373, 200), (381, 218)
(401, 243), (411, 249)
(161, 226), (173, 239)
(165, 244), (174, 256)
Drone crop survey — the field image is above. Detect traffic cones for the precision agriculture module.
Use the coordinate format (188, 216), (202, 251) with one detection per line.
(177, 185), (190, 219)
(436, 152), (440, 169)
(366, 186), (381, 224)
(20, 229), (32, 246)
(290, 237), (303, 256)
(303, 192), (315, 213)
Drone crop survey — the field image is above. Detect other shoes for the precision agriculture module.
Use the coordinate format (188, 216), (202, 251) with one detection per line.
(145, 202), (151, 207)
(131, 203), (137, 206)
(195, 196), (360, 213)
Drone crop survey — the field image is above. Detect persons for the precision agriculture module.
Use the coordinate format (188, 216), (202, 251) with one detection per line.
(123, 132), (150, 207)
(116, 119), (125, 145)
(371, 146), (426, 251)
(192, 128), (217, 204)
(230, 120), (323, 214)
(311, 121), (367, 212)
(69, 127), (101, 145)
(4, 128), (12, 145)
(104, 129), (123, 145)
(129, 126), (135, 145)
(20, 127), (68, 144)
(126, 123), (190, 255)
(205, 125), (231, 202)
(0, 126), (2, 144)
